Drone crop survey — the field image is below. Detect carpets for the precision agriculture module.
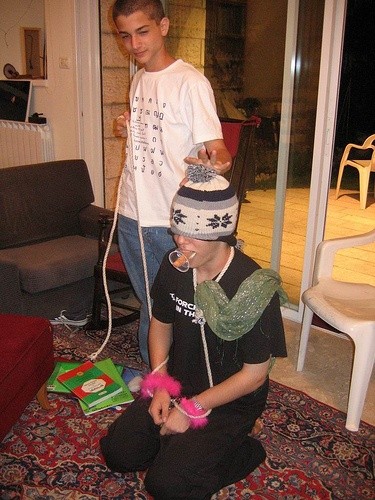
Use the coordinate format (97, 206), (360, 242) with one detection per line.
(0, 304), (375, 500)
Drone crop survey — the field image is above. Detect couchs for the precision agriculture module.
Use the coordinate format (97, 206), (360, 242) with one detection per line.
(0, 158), (119, 320)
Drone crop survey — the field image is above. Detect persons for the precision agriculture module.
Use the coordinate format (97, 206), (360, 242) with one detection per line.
(99, 166), (289, 500)
(242, 97), (273, 141)
(112, 0), (232, 365)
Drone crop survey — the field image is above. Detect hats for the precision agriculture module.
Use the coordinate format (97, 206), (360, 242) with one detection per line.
(167, 165), (240, 246)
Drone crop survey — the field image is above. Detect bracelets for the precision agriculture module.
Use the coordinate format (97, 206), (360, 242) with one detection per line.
(191, 397), (202, 411)
(180, 398), (208, 428)
(139, 370), (181, 401)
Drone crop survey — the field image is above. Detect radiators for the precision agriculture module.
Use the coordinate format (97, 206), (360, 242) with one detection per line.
(0, 119), (55, 170)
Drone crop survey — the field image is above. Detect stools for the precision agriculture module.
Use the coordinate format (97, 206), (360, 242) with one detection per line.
(0, 313), (54, 442)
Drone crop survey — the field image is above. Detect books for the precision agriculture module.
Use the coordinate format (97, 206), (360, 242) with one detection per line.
(78, 358), (135, 415)
(46, 362), (123, 393)
(58, 360), (122, 407)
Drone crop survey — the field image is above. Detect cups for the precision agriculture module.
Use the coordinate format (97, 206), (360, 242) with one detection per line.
(168, 248), (196, 273)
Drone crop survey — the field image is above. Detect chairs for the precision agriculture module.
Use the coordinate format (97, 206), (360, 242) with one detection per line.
(296, 229), (375, 432)
(91, 116), (257, 329)
(335, 134), (375, 209)
